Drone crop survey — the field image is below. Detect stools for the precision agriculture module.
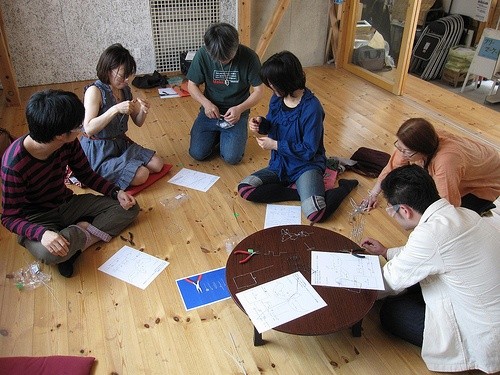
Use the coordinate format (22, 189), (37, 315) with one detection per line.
(440, 46), (475, 88)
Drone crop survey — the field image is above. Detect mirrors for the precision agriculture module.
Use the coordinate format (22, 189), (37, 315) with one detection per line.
(343, 0), (421, 96)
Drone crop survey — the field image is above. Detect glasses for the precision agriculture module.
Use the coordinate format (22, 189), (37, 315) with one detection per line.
(112, 70), (131, 83)
(393, 140), (419, 158)
(386, 204), (400, 217)
(70, 124), (83, 133)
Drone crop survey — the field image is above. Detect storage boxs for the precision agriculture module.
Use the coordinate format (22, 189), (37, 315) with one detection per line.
(352, 19), (405, 71)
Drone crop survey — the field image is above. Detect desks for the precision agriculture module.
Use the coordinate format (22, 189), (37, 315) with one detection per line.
(226, 225), (378, 346)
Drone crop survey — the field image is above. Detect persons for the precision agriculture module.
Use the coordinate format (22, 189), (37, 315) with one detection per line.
(359, 118), (500, 217)
(185, 22), (264, 166)
(0, 89), (141, 277)
(76, 43), (164, 187)
(238, 50), (359, 224)
(359, 164), (500, 375)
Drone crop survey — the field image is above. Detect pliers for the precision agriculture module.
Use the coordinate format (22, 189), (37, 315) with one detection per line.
(186, 274), (202, 294)
(120, 232), (136, 246)
(338, 248), (366, 258)
(234, 251), (259, 263)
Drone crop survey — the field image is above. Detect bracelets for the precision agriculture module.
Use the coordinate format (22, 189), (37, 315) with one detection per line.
(111, 187), (120, 200)
(367, 189), (380, 197)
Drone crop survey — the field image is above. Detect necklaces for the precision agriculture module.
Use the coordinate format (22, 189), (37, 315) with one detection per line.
(221, 61), (232, 86)
(113, 89), (121, 102)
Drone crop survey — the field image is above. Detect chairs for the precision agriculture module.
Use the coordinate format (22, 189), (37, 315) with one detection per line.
(411, 13), (464, 81)
